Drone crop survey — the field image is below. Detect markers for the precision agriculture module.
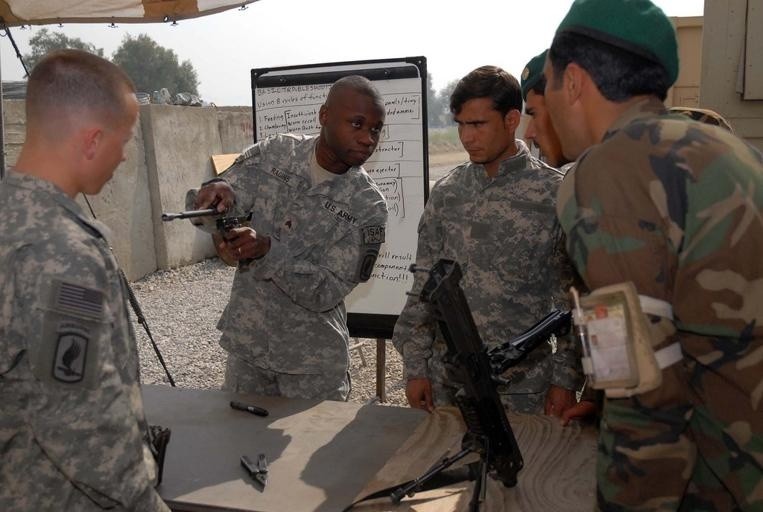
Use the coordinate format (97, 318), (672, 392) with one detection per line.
(231, 400), (269, 418)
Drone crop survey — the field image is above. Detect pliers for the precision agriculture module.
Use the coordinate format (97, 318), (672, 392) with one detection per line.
(239, 454), (268, 487)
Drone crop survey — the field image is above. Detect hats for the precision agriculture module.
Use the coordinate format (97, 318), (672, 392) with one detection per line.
(520, 0), (678, 102)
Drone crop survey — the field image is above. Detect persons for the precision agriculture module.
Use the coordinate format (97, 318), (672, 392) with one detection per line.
(0, 49), (171, 512)
(543, 1), (763, 511)
(520, 48), (606, 429)
(188, 75), (388, 401)
(391, 65), (580, 418)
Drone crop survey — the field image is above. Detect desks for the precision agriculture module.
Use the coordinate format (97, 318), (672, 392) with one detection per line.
(136, 379), (602, 511)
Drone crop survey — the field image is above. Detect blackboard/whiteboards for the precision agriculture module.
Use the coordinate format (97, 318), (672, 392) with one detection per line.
(248, 56), (431, 338)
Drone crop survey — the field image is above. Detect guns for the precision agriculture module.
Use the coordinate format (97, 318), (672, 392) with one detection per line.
(393, 259), (571, 510)
(161, 203), (252, 273)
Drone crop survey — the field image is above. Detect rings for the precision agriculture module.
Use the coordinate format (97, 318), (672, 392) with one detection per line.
(238, 247), (242, 255)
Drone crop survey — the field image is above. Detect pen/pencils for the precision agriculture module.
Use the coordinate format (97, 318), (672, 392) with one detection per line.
(566, 285), (595, 388)
